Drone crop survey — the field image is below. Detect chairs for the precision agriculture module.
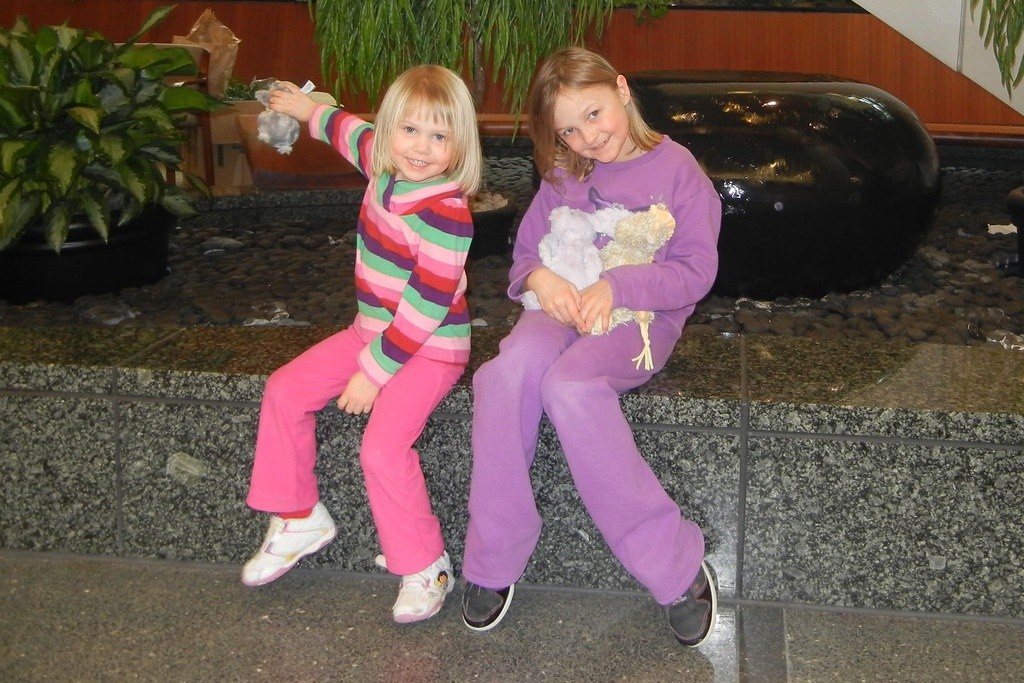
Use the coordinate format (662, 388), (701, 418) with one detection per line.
(113, 41), (214, 188)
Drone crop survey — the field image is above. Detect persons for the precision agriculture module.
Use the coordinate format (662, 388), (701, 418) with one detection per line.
(240, 65), (484, 623)
(461, 49), (717, 648)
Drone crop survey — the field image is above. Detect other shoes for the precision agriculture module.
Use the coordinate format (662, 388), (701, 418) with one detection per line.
(667, 561), (717, 647)
(461, 580), (514, 631)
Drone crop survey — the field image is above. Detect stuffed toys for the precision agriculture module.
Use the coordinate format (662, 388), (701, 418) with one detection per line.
(255, 79), (316, 155)
(523, 202), (677, 363)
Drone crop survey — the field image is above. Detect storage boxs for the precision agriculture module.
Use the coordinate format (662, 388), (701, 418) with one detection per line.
(183, 99), (265, 195)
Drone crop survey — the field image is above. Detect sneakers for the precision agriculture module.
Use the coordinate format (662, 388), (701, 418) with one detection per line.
(392, 550), (455, 623)
(241, 501), (338, 587)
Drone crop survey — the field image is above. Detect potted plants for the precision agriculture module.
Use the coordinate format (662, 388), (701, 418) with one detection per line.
(1, 0), (239, 303)
(307, 0), (675, 263)
(969, 0), (1023, 262)
